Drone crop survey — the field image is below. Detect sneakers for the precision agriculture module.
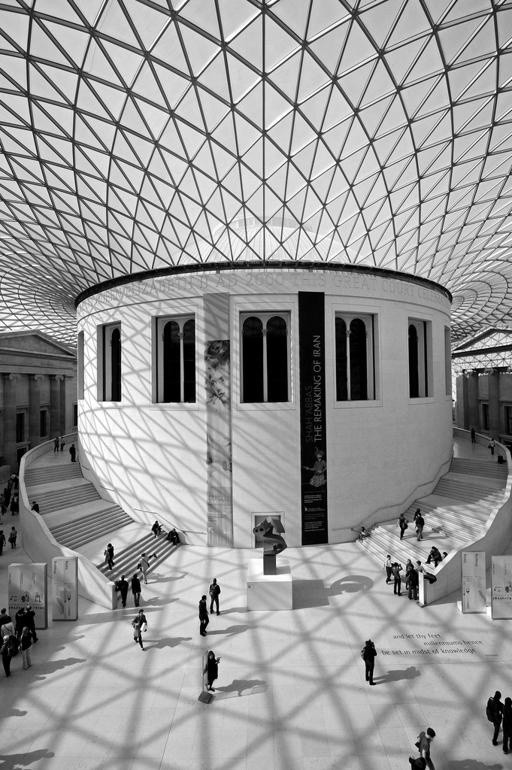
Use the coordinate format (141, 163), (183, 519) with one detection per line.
(6, 664), (31, 679)
(493, 740), (510, 755)
(366, 677), (376, 686)
(386, 578), (420, 601)
(400, 535), (439, 567)
(108, 535), (159, 653)
(194, 608), (228, 692)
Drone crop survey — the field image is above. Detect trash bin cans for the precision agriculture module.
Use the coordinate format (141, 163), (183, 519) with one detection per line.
(498, 456), (503, 464)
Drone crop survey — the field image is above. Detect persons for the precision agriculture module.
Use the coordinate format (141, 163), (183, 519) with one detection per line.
(358, 526), (369, 539)
(152, 521), (162, 537)
(382, 545), (448, 601)
(198, 594), (209, 637)
(167, 529), (180, 546)
(398, 507), (424, 542)
(471, 427), (476, 443)
(0, 604), (40, 678)
(30, 501), (40, 514)
(0, 476), (20, 516)
(69, 444), (77, 462)
(208, 578), (221, 615)
(131, 609), (148, 651)
(365, 640), (378, 685)
(8, 526), (17, 549)
(408, 726), (436, 770)
(104, 543), (115, 570)
(0, 530), (6, 556)
(52, 436), (65, 453)
(486, 691), (512, 756)
(487, 437), (496, 455)
(115, 553), (150, 608)
(203, 650), (221, 692)
